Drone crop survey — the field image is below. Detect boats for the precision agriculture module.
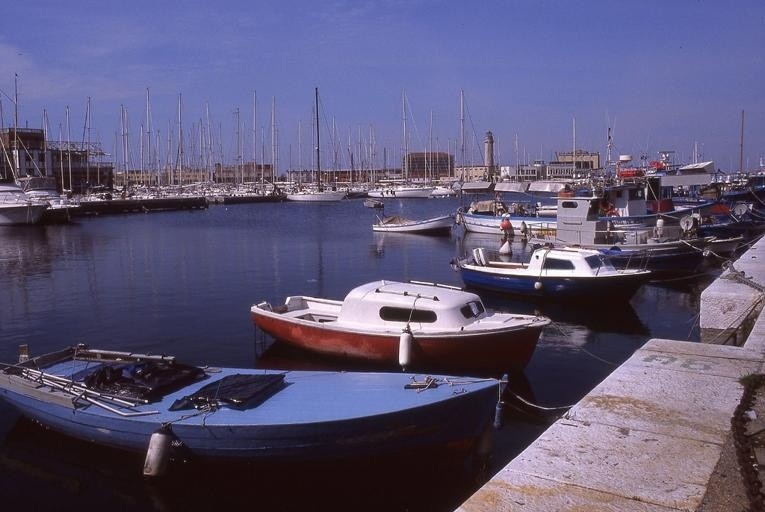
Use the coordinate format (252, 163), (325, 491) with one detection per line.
(453, 244), (652, 304)
(1, 342), (509, 464)
(251, 277), (551, 374)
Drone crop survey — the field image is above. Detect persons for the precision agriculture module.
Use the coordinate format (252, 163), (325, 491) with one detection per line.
(682, 220), (693, 240)
(601, 203), (625, 246)
(652, 225), (657, 238)
(656, 215), (664, 238)
